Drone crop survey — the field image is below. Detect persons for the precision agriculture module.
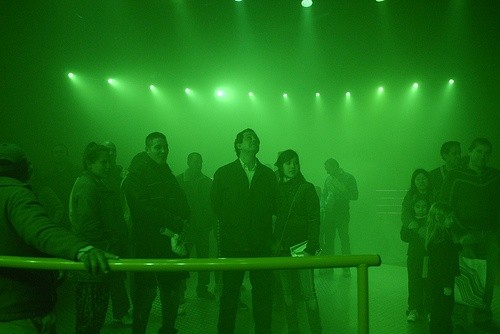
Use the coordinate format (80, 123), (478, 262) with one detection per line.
(426, 201), (467, 334)
(119, 131), (190, 334)
(274, 150), (323, 334)
(321, 157), (359, 279)
(0, 139), (131, 334)
(402, 168), (440, 316)
(400, 195), (435, 323)
(176, 152), (217, 301)
(211, 129), (277, 334)
(441, 138), (500, 334)
(431, 141), (464, 194)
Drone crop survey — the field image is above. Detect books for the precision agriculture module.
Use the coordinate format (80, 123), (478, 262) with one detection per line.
(290, 241), (323, 257)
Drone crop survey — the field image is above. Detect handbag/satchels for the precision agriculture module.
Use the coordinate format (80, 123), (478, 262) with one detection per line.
(271, 235), (284, 257)
(452, 252), (488, 310)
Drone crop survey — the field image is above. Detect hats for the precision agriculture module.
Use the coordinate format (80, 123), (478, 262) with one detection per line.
(0, 143), (32, 171)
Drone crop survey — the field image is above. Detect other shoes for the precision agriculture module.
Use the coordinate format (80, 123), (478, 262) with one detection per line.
(196, 287), (216, 300)
(238, 301), (249, 310)
(158, 327), (178, 334)
(342, 269), (351, 276)
(112, 315), (135, 325)
(407, 310), (421, 321)
